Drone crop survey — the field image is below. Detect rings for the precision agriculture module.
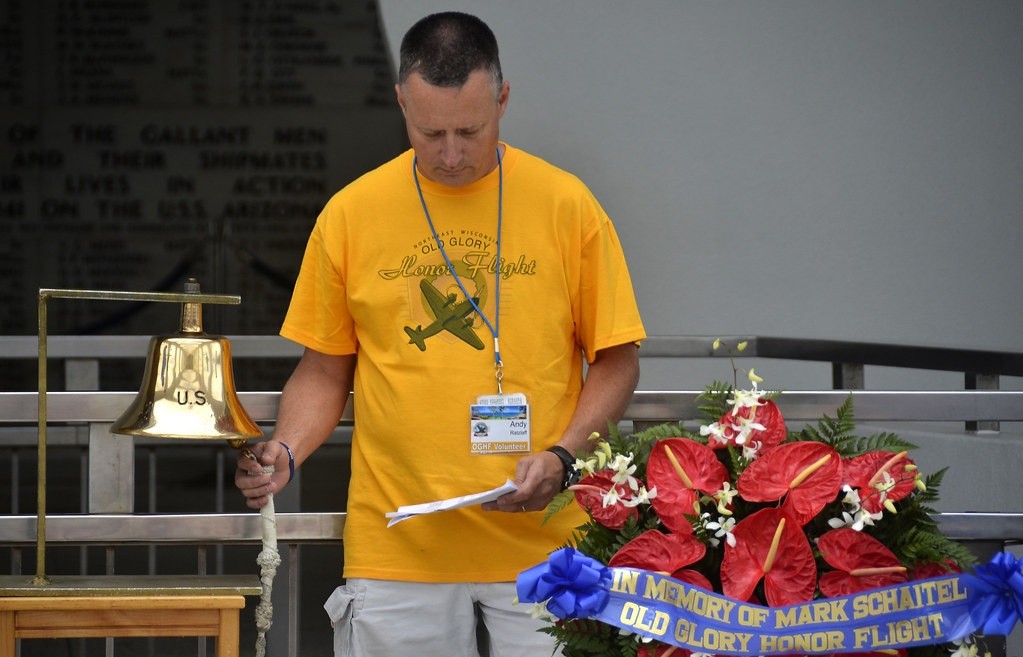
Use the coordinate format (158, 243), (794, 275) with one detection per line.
(522, 505), (526, 512)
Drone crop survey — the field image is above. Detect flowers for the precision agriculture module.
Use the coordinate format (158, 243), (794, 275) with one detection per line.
(953, 551), (1023, 636)
(546, 339), (1005, 657)
(515, 545), (613, 620)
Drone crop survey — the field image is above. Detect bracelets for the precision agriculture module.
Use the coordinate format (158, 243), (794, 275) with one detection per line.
(279, 441), (294, 483)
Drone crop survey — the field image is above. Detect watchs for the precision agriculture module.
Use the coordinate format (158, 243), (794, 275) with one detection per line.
(547, 445), (581, 493)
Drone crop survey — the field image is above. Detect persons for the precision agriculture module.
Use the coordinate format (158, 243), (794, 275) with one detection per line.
(235, 12), (648, 657)
